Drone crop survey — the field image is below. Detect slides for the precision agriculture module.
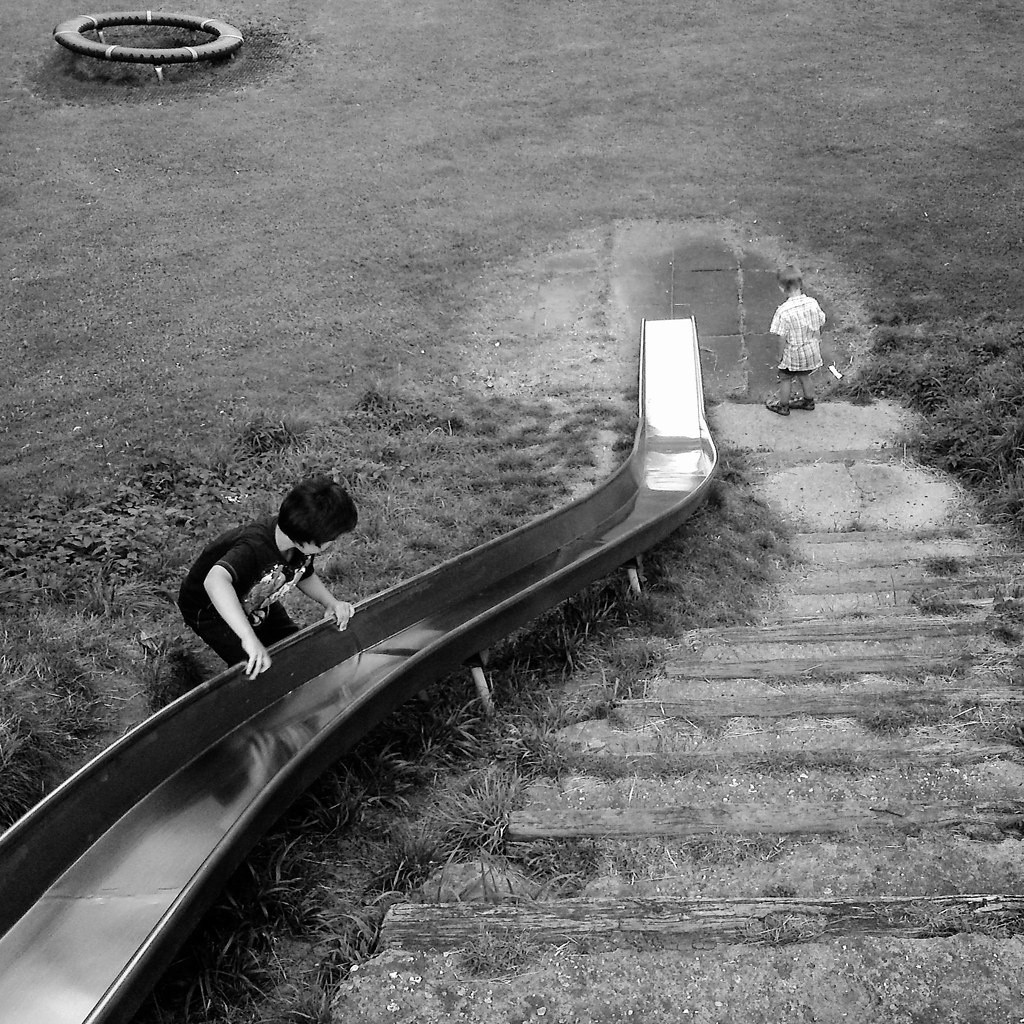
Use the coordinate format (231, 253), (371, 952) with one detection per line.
(1, 315), (719, 1024)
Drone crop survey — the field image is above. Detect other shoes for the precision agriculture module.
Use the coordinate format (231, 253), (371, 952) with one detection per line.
(767, 399), (790, 415)
(789, 397), (815, 410)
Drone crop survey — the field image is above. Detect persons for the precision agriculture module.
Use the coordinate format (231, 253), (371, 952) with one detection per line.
(177, 476), (358, 680)
(765, 265), (826, 416)
